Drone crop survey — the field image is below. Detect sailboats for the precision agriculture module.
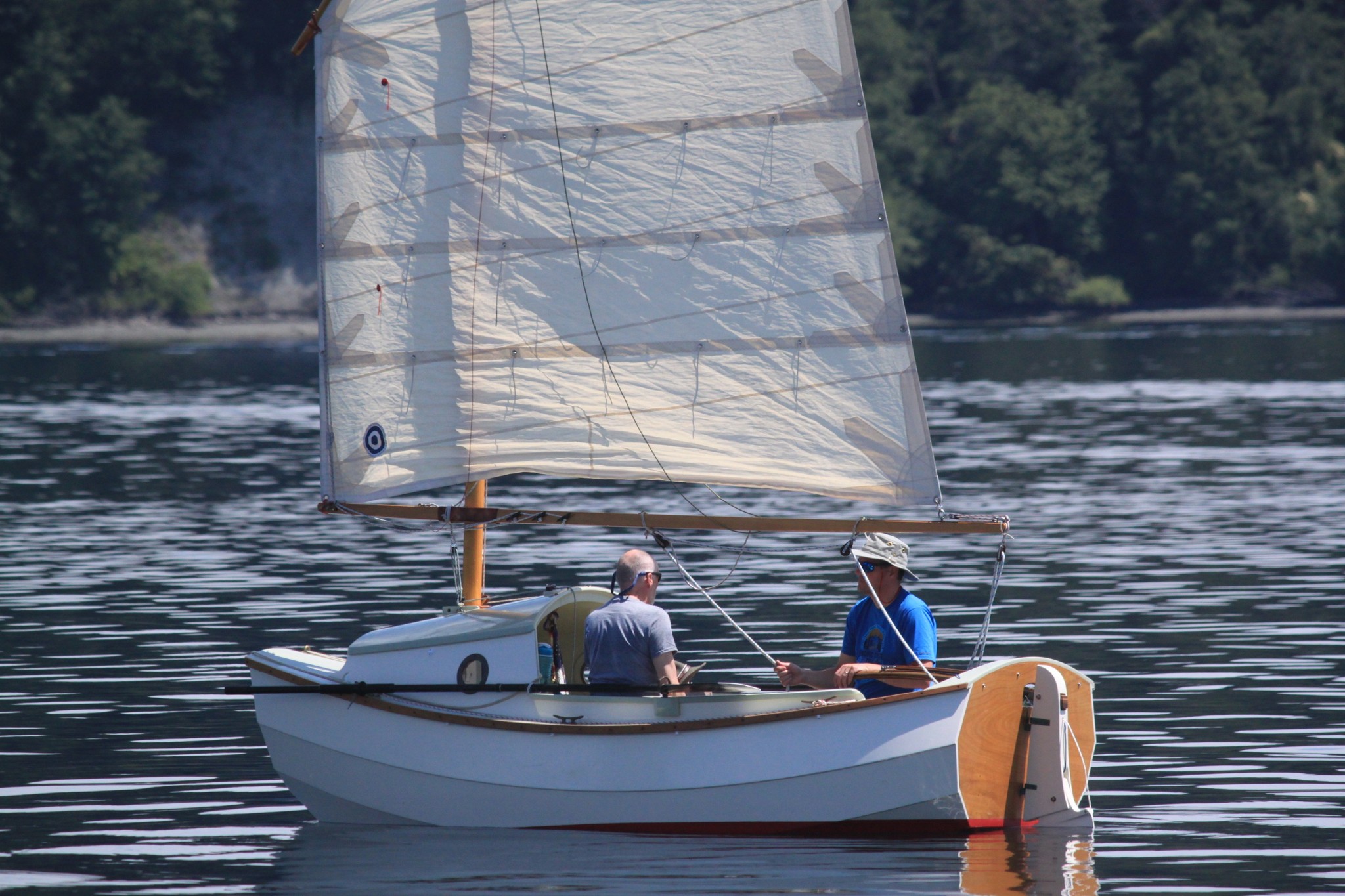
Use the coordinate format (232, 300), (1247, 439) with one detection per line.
(217, 0), (1098, 841)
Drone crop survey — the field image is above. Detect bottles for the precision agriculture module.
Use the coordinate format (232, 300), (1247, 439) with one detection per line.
(538, 642), (553, 686)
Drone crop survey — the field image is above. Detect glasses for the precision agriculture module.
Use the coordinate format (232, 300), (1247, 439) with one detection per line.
(638, 572), (662, 583)
(857, 560), (893, 573)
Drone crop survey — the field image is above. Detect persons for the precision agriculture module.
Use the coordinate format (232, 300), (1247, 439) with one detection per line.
(773, 533), (936, 699)
(585, 550), (686, 698)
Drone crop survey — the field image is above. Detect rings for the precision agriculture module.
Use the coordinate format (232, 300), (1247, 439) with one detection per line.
(844, 672), (849, 674)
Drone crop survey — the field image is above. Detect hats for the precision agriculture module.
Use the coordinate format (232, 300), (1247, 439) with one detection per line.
(849, 532), (919, 581)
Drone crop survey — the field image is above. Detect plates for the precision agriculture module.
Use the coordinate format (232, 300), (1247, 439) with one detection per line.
(717, 682), (761, 693)
